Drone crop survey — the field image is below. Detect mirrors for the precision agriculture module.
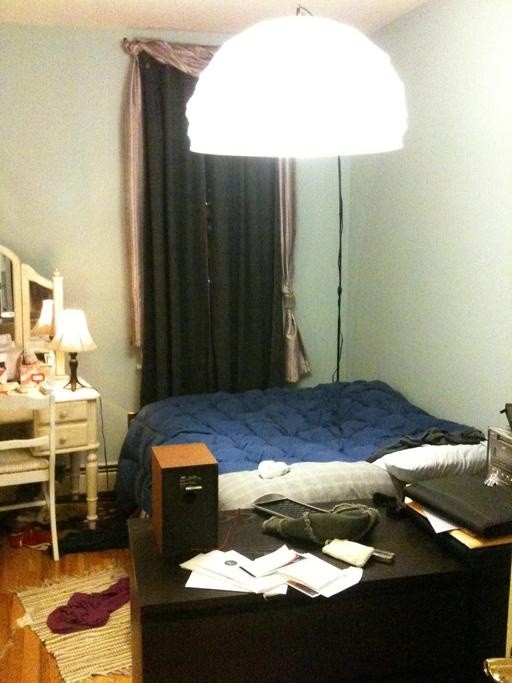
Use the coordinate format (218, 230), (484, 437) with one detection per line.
(29, 281), (52, 358)
(0, 254), (15, 342)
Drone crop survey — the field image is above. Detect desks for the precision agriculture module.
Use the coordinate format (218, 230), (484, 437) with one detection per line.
(0, 246), (102, 530)
(128, 500), (512, 683)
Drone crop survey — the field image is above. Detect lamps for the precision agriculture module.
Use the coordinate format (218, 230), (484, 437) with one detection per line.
(31, 299), (53, 337)
(48, 308), (99, 392)
(185, 0), (411, 160)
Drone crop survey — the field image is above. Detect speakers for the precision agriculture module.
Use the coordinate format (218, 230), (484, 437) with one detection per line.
(151, 442), (219, 557)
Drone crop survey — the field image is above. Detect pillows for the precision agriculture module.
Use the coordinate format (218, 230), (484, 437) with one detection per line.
(244, 461), (401, 509)
(377, 443), (486, 482)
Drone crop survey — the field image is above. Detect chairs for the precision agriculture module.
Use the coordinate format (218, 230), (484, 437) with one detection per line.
(0, 395), (60, 562)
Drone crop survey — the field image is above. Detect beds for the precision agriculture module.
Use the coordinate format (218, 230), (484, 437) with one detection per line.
(114, 381), (483, 518)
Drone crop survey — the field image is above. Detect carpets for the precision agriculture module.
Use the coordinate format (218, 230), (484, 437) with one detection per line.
(12, 559), (132, 683)
